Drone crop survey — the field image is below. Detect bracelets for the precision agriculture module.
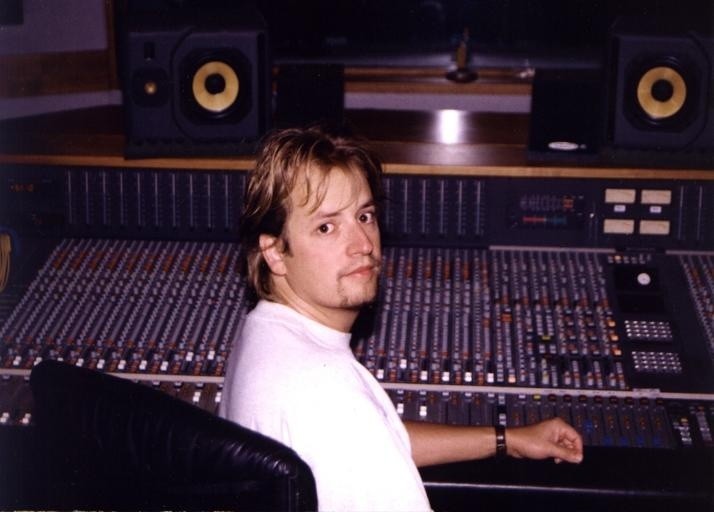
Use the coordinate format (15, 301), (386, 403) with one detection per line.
(495, 425), (508, 457)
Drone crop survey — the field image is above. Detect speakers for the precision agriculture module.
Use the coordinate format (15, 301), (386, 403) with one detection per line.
(530, 66), (609, 155)
(126, 30), (271, 153)
(276, 62), (345, 137)
(609, 27), (714, 149)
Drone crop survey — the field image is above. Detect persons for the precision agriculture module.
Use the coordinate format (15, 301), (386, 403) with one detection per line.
(217, 126), (585, 512)
